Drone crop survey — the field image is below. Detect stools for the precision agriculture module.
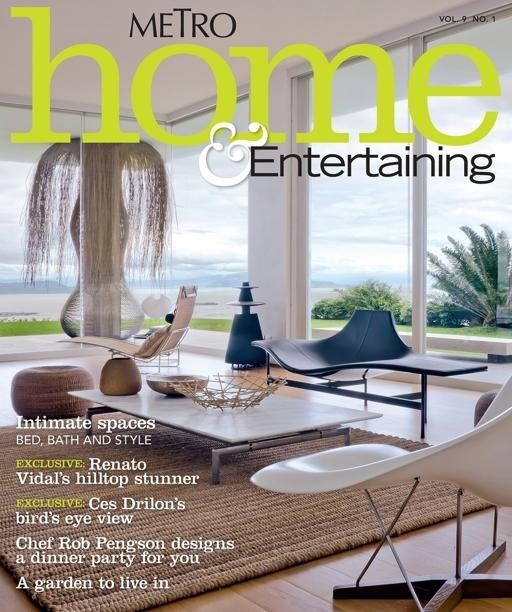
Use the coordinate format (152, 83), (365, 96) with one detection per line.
(474, 392), (497, 424)
(11, 365), (95, 421)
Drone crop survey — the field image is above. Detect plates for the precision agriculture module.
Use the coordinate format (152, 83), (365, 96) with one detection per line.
(146, 376), (209, 398)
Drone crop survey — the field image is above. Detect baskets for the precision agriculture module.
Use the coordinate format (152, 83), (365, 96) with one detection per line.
(171, 372), (287, 412)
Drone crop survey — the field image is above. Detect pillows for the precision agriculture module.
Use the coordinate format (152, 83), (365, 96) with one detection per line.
(133, 326), (170, 357)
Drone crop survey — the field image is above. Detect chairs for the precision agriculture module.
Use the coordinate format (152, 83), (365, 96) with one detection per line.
(250, 377), (511, 612)
(56, 284), (198, 376)
(251, 309), (490, 439)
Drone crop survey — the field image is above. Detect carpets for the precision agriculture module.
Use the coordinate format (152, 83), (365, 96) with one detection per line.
(0, 410), (503, 612)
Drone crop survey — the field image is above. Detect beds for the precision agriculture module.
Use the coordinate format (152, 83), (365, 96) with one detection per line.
(67, 380), (383, 485)
